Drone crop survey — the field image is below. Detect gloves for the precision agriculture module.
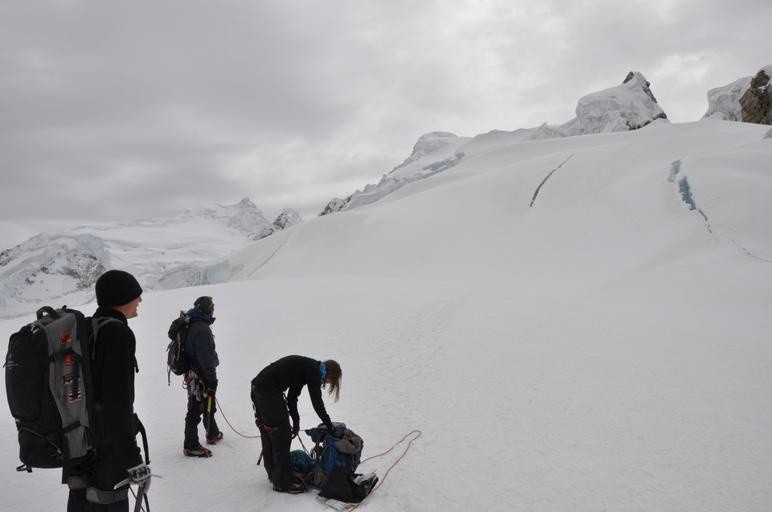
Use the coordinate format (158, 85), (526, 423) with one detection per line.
(202, 385), (216, 402)
(125, 464), (152, 494)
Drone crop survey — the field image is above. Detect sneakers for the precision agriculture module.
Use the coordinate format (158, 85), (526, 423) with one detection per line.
(205, 432), (223, 444)
(272, 481), (306, 494)
(183, 443), (212, 457)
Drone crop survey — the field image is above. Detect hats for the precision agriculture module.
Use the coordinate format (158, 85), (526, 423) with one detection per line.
(94, 269), (144, 308)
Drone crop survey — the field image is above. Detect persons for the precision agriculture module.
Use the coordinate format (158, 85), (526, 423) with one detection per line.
(175, 295), (225, 458)
(249, 354), (344, 493)
(60, 269), (152, 512)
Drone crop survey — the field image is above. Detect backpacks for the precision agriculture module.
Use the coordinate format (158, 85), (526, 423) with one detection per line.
(2, 305), (102, 474)
(287, 411), (380, 503)
(165, 333), (195, 375)
